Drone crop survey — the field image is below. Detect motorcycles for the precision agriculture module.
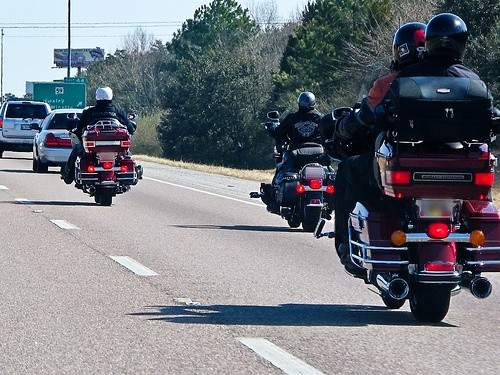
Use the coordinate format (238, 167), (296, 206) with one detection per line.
(249, 106), (336, 234)
(59, 104), (144, 207)
(313, 54), (500, 325)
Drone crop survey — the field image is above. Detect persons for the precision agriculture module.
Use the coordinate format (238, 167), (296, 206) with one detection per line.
(64, 86), (137, 185)
(334, 12), (500, 276)
(267, 91), (332, 185)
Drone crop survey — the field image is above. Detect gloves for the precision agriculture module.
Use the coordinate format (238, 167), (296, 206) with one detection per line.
(263, 122), (275, 135)
(332, 117), (354, 148)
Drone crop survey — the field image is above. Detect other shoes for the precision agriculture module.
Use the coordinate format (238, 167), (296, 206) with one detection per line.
(133, 165), (143, 185)
(266, 203), (279, 213)
(60, 166), (73, 185)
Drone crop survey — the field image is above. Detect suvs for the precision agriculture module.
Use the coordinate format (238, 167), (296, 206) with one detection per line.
(0, 100), (53, 159)
(29, 106), (94, 173)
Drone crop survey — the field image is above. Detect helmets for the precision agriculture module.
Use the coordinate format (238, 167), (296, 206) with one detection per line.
(96, 86), (113, 101)
(425, 13), (468, 49)
(297, 92), (315, 108)
(392, 22), (427, 62)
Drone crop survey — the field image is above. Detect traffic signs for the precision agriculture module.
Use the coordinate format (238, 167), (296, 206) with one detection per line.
(33, 77), (86, 111)
